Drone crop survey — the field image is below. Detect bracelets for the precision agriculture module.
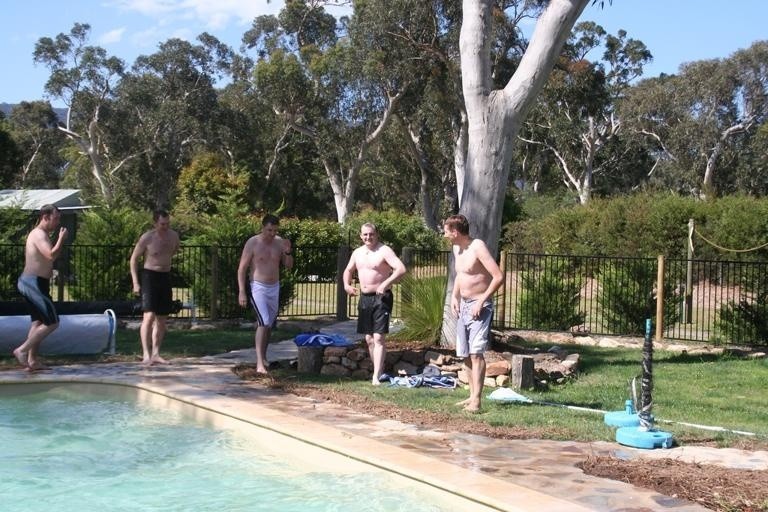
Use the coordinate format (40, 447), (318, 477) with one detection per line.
(284, 251), (290, 256)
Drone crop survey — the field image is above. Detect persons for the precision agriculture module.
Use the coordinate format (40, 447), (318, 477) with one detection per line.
(129, 208), (180, 366)
(442, 214), (505, 413)
(12, 203), (69, 373)
(236, 213), (294, 376)
(341, 221), (406, 388)
